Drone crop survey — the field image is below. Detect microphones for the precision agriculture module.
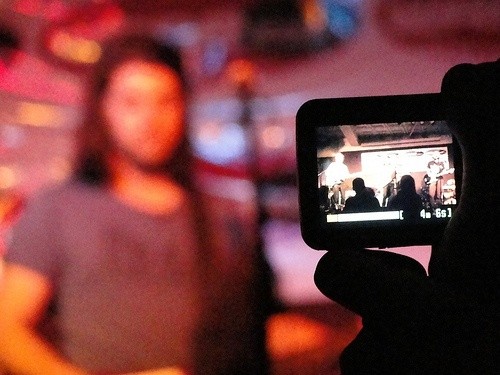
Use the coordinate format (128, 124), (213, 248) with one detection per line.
(229, 59), (260, 101)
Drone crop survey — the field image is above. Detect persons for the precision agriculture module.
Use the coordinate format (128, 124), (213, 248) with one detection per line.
(424, 161), (456, 204)
(314, 56), (499, 375)
(365, 187), (380, 209)
(320, 185), (330, 212)
(344, 178), (380, 210)
(1, 29), (258, 375)
(323, 153), (351, 210)
(387, 175), (423, 208)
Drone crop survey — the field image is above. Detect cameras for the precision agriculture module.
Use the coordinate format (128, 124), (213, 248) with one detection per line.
(295, 91), (463, 250)
